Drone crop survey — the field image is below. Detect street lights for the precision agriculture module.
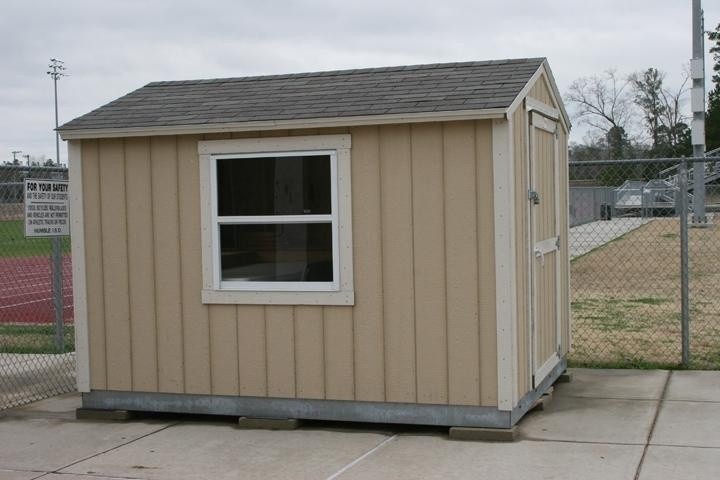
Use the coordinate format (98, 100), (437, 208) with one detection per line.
(10, 150), (23, 202)
(47, 56), (69, 180)
(23, 153), (31, 178)
